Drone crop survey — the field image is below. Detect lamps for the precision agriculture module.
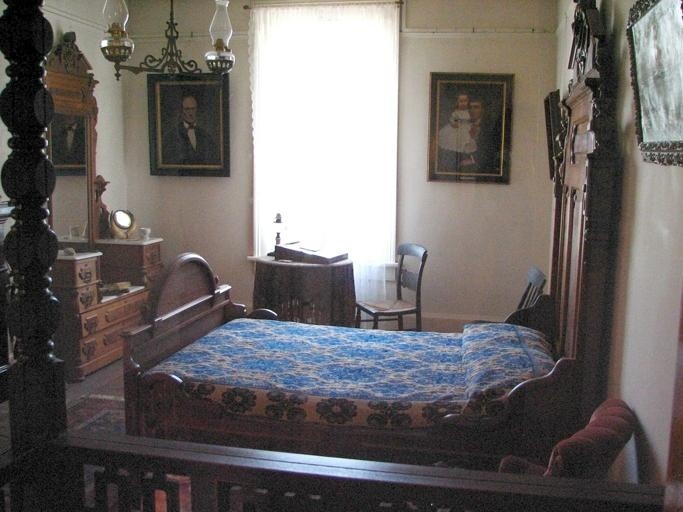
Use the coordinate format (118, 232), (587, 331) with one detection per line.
(100, 0), (236, 80)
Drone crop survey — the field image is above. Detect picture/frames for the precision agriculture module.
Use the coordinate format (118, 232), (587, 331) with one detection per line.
(147, 73), (231, 177)
(543, 88), (563, 179)
(48, 116), (86, 176)
(428, 71), (514, 185)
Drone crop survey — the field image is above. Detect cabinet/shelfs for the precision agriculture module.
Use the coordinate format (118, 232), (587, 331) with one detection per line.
(35, 234), (164, 380)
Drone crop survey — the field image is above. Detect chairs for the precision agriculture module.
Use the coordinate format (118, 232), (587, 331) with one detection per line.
(516, 266), (546, 312)
(356, 243), (428, 331)
(498, 398), (637, 479)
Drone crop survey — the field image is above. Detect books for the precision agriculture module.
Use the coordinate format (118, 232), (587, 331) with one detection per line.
(273, 239), (350, 264)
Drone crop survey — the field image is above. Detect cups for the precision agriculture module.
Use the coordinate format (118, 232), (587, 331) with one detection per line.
(139, 227), (151, 241)
(69, 225), (82, 240)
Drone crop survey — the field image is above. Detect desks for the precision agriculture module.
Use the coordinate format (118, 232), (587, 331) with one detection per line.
(253, 255), (356, 329)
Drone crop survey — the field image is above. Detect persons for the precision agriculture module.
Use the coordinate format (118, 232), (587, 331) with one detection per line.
(436, 90), (478, 152)
(459, 97), (501, 174)
(161, 91), (220, 165)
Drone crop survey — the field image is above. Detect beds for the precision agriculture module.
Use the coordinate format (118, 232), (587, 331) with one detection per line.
(121, 252), (617, 472)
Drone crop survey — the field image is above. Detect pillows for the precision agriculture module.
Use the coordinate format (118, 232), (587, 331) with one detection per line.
(462, 322), (553, 397)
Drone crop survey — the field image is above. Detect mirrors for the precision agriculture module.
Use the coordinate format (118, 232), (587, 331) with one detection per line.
(626, 0), (683, 166)
(41, 31), (109, 255)
(110, 208), (138, 239)
